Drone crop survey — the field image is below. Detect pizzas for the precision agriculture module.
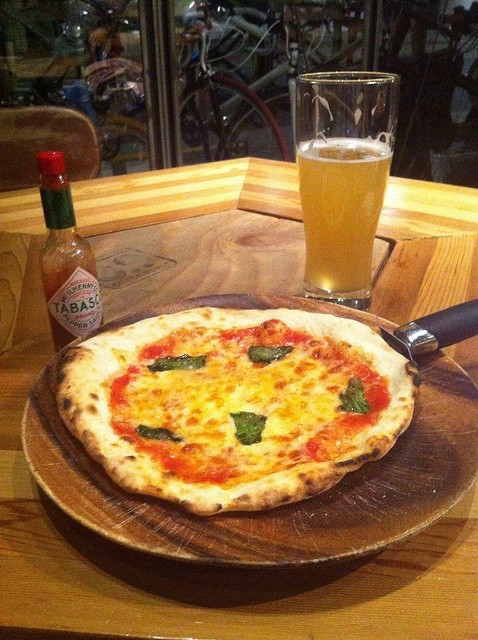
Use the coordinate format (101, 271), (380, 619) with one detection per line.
(54, 305), (415, 517)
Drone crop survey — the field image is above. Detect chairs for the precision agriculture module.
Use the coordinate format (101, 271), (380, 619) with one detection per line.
(0, 107), (100, 191)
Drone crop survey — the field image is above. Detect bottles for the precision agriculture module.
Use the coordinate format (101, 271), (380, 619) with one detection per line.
(291, 72), (397, 306)
(31, 146), (104, 350)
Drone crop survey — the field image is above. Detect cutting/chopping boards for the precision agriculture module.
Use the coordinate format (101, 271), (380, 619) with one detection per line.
(21, 295), (478, 571)
